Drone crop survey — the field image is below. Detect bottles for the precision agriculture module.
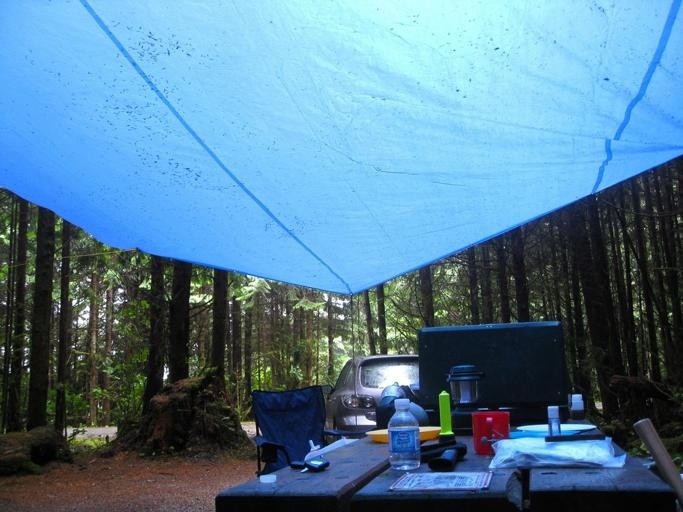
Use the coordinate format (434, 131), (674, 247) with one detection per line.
(570, 393), (586, 423)
(546, 406), (561, 437)
(388, 397), (421, 471)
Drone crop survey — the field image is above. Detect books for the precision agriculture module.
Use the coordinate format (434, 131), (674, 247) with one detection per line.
(390, 471), (492, 492)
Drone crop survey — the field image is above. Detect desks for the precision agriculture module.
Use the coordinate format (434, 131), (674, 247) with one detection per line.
(212, 424), (679, 511)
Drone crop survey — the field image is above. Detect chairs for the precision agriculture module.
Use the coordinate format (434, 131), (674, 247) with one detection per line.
(250, 384), (343, 475)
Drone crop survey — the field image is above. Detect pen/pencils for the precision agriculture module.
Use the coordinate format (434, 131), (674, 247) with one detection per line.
(544, 434), (605, 442)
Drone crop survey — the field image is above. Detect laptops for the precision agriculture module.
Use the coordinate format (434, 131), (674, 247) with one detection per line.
(417, 320), (570, 428)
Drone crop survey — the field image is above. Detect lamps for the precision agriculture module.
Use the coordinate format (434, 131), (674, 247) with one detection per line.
(443, 360), (485, 436)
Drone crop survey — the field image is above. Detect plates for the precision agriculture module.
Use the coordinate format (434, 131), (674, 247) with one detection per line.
(364, 426), (444, 444)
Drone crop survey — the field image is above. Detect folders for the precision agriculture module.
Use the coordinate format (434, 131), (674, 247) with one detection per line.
(508, 430), (580, 438)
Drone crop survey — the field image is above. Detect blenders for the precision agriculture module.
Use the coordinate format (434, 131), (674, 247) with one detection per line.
(446, 364), (487, 436)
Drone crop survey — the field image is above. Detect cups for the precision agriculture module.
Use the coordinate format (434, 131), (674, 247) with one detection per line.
(471, 410), (511, 454)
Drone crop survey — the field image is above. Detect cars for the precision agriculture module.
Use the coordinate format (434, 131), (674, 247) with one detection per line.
(319, 354), (418, 443)
